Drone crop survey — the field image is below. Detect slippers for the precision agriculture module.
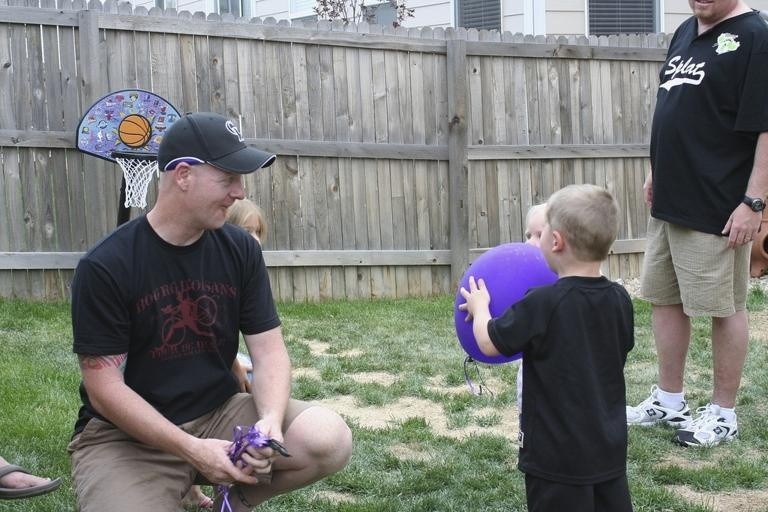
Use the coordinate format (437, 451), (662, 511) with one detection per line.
(0, 464), (62, 498)
(196, 496), (213, 509)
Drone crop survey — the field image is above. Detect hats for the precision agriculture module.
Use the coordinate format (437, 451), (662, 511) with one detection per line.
(158, 112), (276, 174)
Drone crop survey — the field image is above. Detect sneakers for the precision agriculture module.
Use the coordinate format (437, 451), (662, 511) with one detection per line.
(627, 389), (694, 426)
(671, 404), (739, 447)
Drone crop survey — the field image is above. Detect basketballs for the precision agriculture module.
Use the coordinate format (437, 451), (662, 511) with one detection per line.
(118, 114), (152, 148)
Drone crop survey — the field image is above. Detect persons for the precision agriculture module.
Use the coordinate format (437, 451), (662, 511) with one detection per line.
(515, 202), (546, 419)
(626, 0), (767, 448)
(66, 112), (353, 512)
(226, 198), (269, 392)
(458, 182), (635, 511)
(0, 455), (62, 499)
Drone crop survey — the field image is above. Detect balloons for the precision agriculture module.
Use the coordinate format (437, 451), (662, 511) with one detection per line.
(455, 242), (558, 365)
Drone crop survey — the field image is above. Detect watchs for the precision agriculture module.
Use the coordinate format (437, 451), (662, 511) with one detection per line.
(742, 196), (767, 212)
